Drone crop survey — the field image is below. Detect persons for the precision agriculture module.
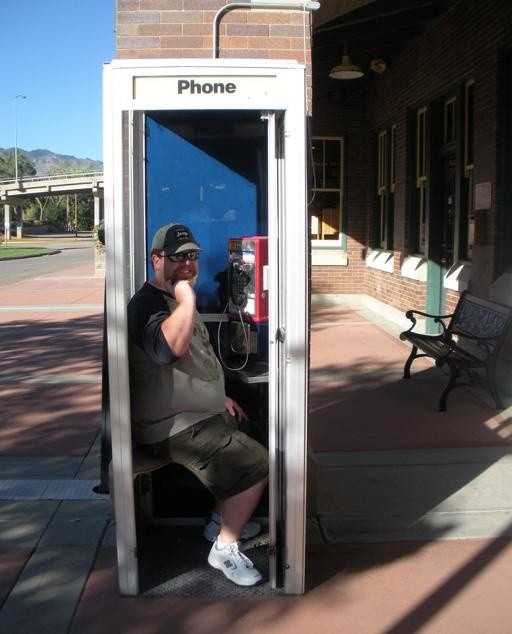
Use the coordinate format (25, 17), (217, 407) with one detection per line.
(126, 221), (271, 588)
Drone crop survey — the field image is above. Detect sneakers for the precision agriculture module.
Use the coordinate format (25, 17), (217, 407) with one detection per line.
(206, 539), (263, 587)
(203, 513), (262, 543)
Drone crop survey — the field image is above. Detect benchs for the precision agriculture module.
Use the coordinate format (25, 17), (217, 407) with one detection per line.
(399, 289), (511, 411)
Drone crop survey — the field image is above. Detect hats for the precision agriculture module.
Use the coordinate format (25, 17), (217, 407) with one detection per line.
(150, 223), (204, 255)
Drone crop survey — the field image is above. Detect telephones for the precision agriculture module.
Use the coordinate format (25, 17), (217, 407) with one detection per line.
(227, 236), (268, 326)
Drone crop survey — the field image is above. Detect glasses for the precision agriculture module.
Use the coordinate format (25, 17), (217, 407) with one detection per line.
(158, 251), (201, 261)
